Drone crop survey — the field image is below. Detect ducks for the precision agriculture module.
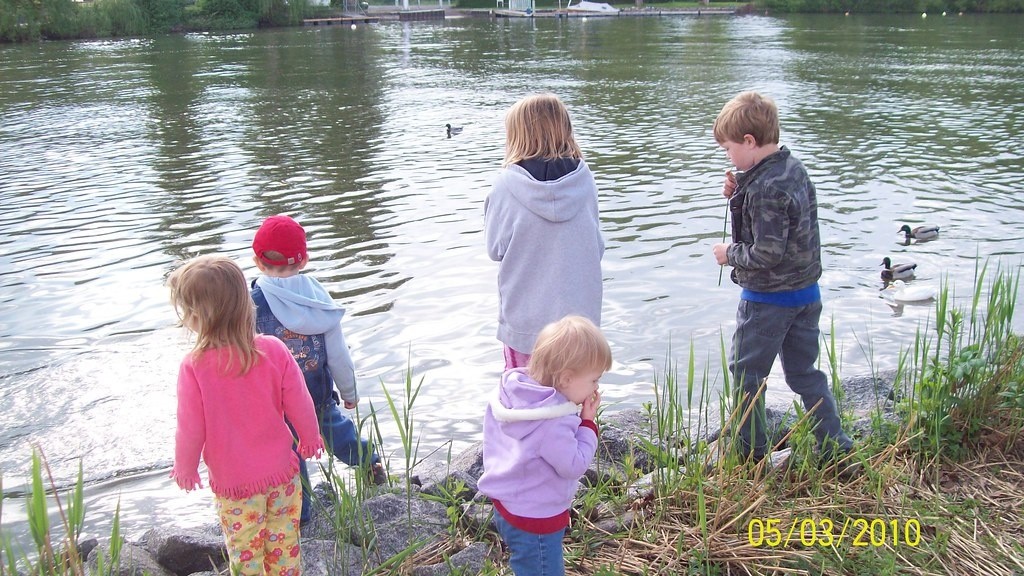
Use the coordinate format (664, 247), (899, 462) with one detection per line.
(444, 124), (464, 133)
(886, 279), (938, 302)
(897, 225), (940, 239)
(879, 257), (918, 279)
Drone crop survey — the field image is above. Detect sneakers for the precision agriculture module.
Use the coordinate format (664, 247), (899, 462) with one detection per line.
(368, 462), (387, 485)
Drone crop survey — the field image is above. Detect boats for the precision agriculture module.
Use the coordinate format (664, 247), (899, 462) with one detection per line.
(558, 0), (621, 17)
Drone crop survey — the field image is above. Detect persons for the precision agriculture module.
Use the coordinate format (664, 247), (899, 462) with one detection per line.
(474, 311), (620, 575)
(481, 91), (608, 374)
(243, 218), (390, 529)
(709, 93), (865, 490)
(159, 251), (326, 576)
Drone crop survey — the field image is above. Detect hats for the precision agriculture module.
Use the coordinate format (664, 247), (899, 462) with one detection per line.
(251, 215), (306, 266)
